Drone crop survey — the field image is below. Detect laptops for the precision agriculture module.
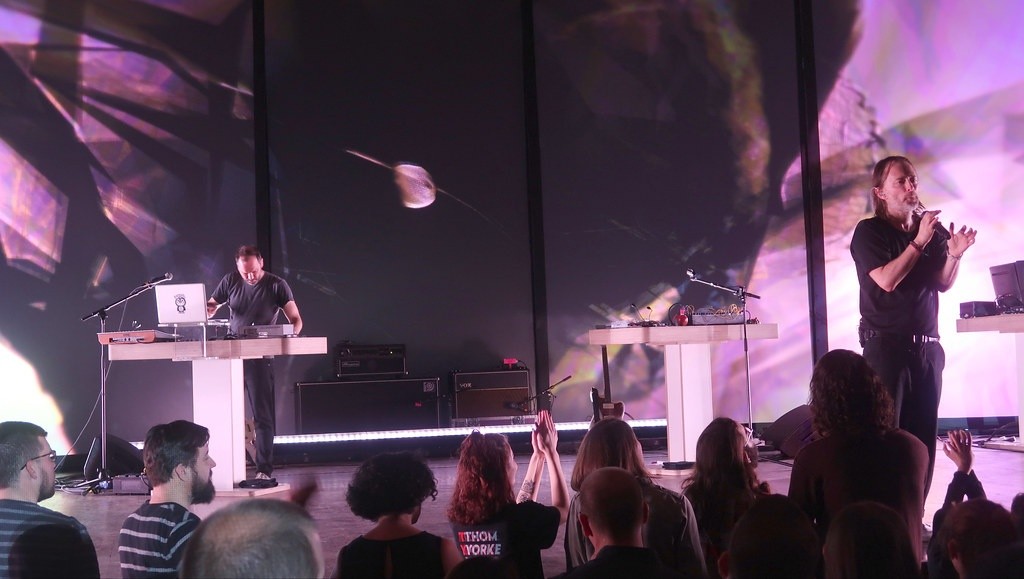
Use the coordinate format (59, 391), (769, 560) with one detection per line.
(154, 283), (228, 323)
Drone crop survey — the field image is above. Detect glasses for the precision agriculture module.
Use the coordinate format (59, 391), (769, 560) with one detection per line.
(742, 425), (753, 447)
(21, 450), (56, 471)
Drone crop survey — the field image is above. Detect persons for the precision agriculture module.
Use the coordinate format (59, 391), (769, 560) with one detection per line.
(717, 492), (822, 579)
(117, 419), (214, 579)
(563, 417), (710, 579)
(848, 156), (978, 532)
(447, 409), (569, 579)
(549, 468), (691, 579)
(787, 348), (932, 570)
(330, 452), (462, 579)
(925, 425), (1024, 579)
(449, 554), (511, 579)
(822, 500), (926, 579)
(679, 416), (772, 579)
(183, 497), (326, 579)
(206, 245), (303, 479)
(0, 420), (102, 579)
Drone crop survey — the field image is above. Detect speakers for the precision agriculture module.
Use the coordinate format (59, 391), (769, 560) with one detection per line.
(760, 404), (825, 458)
(83, 433), (145, 480)
(335, 343), (407, 382)
(295, 377), (441, 435)
(448, 368), (531, 419)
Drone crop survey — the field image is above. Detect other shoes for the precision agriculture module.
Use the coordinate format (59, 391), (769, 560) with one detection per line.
(256, 472), (271, 480)
(921, 520), (933, 540)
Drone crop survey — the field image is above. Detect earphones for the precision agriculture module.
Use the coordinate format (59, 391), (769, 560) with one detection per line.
(878, 191), (884, 196)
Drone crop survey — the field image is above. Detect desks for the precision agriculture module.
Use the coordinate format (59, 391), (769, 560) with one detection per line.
(107, 336), (328, 497)
(589, 323), (778, 476)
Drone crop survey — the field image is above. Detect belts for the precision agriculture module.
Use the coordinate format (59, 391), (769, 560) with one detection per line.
(863, 329), (940, 345)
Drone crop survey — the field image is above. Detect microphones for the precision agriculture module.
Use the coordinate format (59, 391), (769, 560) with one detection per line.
(141, 272), (174, 287)
(920, 211), (951, 240)
(509, 402), (529, 412)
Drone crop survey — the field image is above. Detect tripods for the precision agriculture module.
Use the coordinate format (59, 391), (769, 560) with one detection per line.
(73, 285), (157, 496)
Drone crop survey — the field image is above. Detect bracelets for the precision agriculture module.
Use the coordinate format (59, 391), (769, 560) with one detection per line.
(946, 250), (963, 260)
(909, 240), (929, 257)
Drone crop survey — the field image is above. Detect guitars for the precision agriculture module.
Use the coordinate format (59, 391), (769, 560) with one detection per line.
(588, 323), (625, 430)
